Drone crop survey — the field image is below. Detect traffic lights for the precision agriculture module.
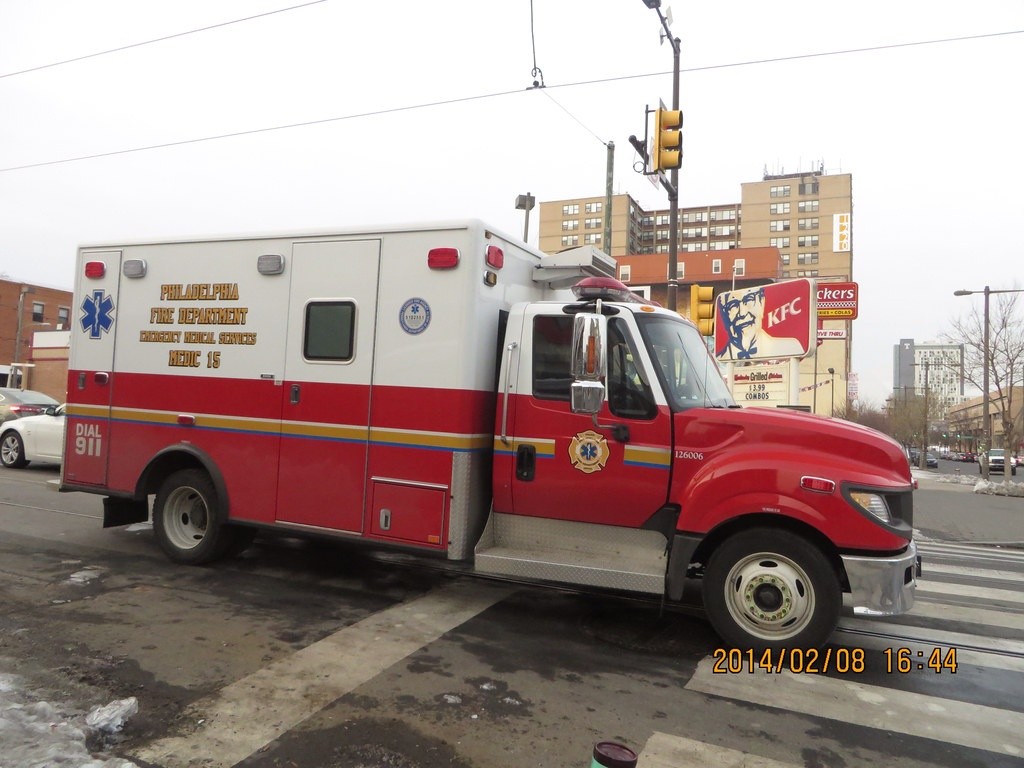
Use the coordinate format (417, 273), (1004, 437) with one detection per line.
(651, 105), (684, 173)
(690, 282), (717, 339)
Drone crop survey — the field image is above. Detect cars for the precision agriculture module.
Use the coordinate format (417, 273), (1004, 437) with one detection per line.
(908, 450), (1024, 476)
(0, 387), (61, 425)
(0, 403), (68, 470)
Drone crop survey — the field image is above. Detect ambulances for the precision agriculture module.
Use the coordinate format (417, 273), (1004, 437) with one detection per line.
(49, 212), (924, 675)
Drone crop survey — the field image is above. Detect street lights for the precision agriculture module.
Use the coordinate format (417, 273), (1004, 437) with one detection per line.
(954, 287), (1024, 479)
(906, 363), (961, 468)
(514, 191), (536, 243)
(885, 399), (921, 440)
(892, 386), (931, 412)
